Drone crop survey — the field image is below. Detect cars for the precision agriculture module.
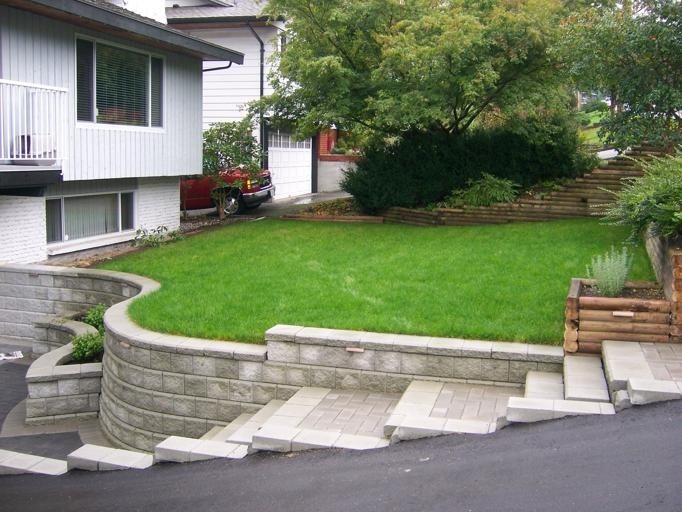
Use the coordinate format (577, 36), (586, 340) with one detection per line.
(180, 147), (278, 221)
